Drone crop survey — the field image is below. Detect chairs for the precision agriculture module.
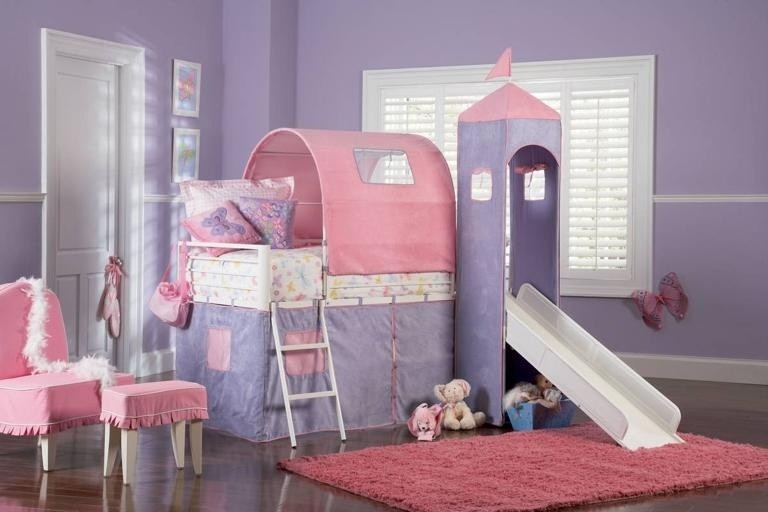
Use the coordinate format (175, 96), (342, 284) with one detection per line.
(0, 276), (135, 472)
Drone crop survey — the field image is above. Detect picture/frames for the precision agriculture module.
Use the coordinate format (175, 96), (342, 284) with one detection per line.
(172, 127), (200, 184)
(172, 58), (202, 119)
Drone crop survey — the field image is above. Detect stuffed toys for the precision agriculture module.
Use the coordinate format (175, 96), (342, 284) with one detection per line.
(408, 403), (442, 441)
(434, 378), (486, 430)
(503, 374), (562, 409)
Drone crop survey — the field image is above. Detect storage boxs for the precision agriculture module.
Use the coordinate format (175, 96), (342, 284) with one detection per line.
(506, 397), (575, 432)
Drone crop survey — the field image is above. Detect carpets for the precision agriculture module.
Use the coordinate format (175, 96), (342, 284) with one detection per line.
(273, 419), (768, 511)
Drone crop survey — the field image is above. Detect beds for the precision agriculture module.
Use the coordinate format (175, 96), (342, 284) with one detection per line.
(177, 126), (457, 310)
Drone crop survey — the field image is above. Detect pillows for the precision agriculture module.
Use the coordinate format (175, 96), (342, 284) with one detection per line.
(180, 198), (262, 258)
(237, 196), (299, 251)
(178, 175), (295, 218)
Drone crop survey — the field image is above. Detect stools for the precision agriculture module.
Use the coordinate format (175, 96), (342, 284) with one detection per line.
(98, 379), (211, 485)
